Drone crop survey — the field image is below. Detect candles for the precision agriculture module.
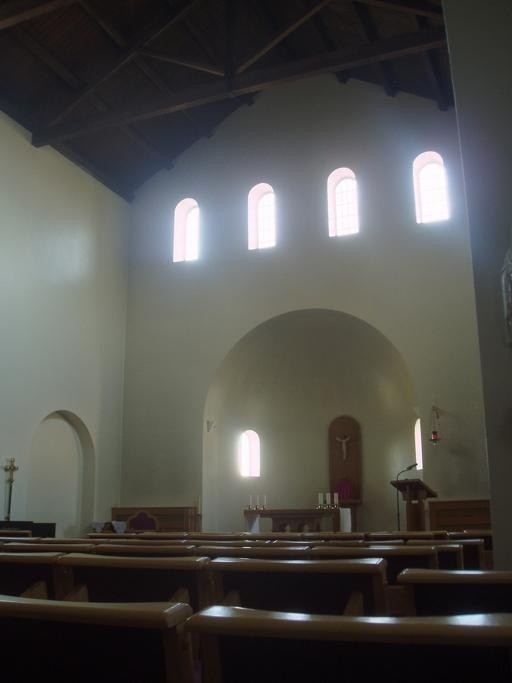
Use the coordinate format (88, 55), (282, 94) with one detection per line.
(249, 495), (267, 505)
(318, 493), (338, 504)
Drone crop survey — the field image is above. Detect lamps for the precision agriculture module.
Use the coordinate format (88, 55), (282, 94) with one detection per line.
(428, 407), (441, 448)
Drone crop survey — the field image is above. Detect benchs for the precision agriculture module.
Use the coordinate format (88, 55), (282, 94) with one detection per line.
(1, 529), (511, 682)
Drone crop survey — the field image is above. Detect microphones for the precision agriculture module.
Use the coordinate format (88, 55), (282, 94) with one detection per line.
(396, 464), (418, 531)
